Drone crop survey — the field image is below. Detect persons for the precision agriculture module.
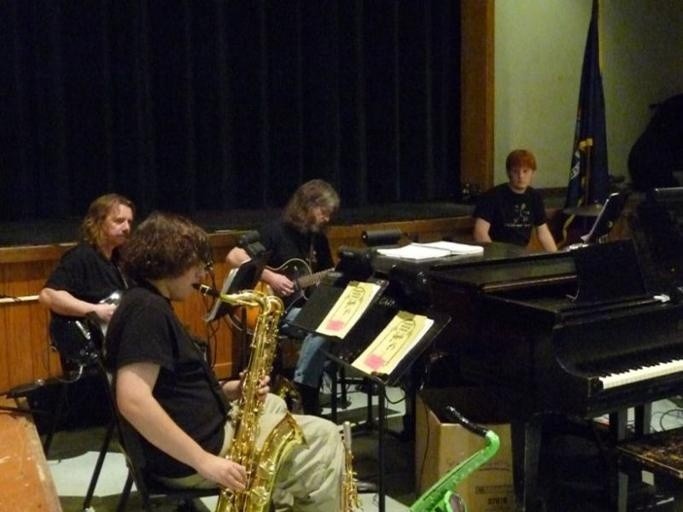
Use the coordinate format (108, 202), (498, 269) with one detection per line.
(36, 194), (137, 381)
(225, 176), (347, 415)
(473, 150), (560, 252)
(100, 213), (360, 510)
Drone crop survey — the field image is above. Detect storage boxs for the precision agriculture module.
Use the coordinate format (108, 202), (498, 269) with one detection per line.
(415, 388), (519, 512)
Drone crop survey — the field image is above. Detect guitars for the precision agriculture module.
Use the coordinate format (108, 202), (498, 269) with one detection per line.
(48, 261), (213, 368)
(225, 257), (336, 336)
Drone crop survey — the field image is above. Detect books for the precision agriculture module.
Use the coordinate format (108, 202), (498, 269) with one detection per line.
(374, 239), (483, 260)
(579, 191), (620, 243)
(350, 309), (434, 381)
(205, 266), (237, 323)
(318, 280), (380, 340)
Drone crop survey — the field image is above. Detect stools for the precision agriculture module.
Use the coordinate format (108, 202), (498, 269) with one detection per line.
(613, 423), (681, 511)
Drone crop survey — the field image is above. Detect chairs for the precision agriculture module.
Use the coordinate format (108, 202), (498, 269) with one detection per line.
(79, 352), (230, 511)
(40, 307), (117, 457)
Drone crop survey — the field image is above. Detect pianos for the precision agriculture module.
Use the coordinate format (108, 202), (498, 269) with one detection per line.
(406, 238), (683, 511)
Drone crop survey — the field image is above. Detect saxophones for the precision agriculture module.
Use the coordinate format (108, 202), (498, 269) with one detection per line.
(191, 283), (311, 512)
(407, 406), (501, 512)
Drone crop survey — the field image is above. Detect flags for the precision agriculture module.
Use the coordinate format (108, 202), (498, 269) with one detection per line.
(563, 0), (612, 202)
